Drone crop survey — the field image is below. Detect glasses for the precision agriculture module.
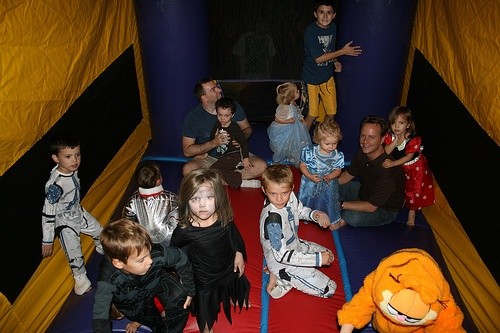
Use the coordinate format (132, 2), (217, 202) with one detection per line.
(211, 85), (221, 92)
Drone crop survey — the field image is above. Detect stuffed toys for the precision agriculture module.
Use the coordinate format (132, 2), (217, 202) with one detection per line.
(336, 247), (467, 333)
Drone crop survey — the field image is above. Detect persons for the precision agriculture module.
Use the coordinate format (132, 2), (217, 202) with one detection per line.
(91, 218), (195, 333)
(381, 106), (435, 226)
(259, 163), (337, 300)
(180, 76), (268, 186)
(202, 96), (261, 188)
(301, 0), (363, 134)
(266, 81), (312, 165)
(121, 160), (180, 244)
(171, 168), (250, 333)
(328, 115), (404, 230)
(41, 131), (105, 296)
(295, 116), (344, 225)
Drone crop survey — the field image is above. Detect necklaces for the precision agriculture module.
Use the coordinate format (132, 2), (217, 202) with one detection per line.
(363, 157), (377, 168)
